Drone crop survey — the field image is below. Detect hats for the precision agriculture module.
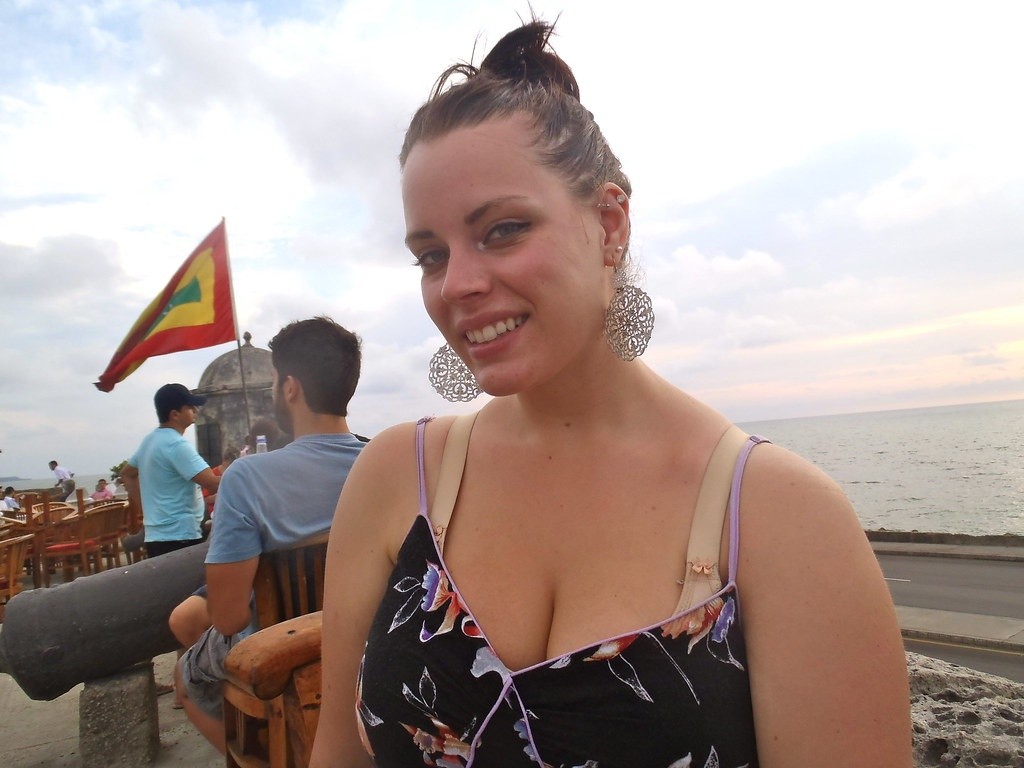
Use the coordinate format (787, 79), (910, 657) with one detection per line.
(154, 383), (208, 408)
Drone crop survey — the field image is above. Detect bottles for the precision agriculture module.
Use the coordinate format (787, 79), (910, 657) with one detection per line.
(256, 435), (268, 453)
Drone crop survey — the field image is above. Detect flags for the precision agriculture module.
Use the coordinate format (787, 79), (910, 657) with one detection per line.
(92, 219), (240, 393)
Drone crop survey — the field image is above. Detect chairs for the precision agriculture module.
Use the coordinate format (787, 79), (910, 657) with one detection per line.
(223, 609), (324, 755)
(0, 487), (148, 627)
(220, 526), (332, 768)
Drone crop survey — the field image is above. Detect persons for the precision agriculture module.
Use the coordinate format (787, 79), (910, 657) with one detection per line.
(93, 484), (111, 500)
(0, 486), (4, 501)
(120, 382), (222, 561)
(60, 480), (77, 503)
(204, 446), (239, 519)
(91, 479), (113, 500)
(242, 331), (254, 348)
(174, 314), (372, 757)
(4, 486), (19, 509)
(240, 420), (289, 463)
(48, 461), (75, 492)
(306, 1), (910, 768)
(169, 581), (259, 650)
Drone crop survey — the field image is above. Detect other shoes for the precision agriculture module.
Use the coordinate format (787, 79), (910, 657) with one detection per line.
(154, 681), (174, 695)
(169, 692), (185, 708)
